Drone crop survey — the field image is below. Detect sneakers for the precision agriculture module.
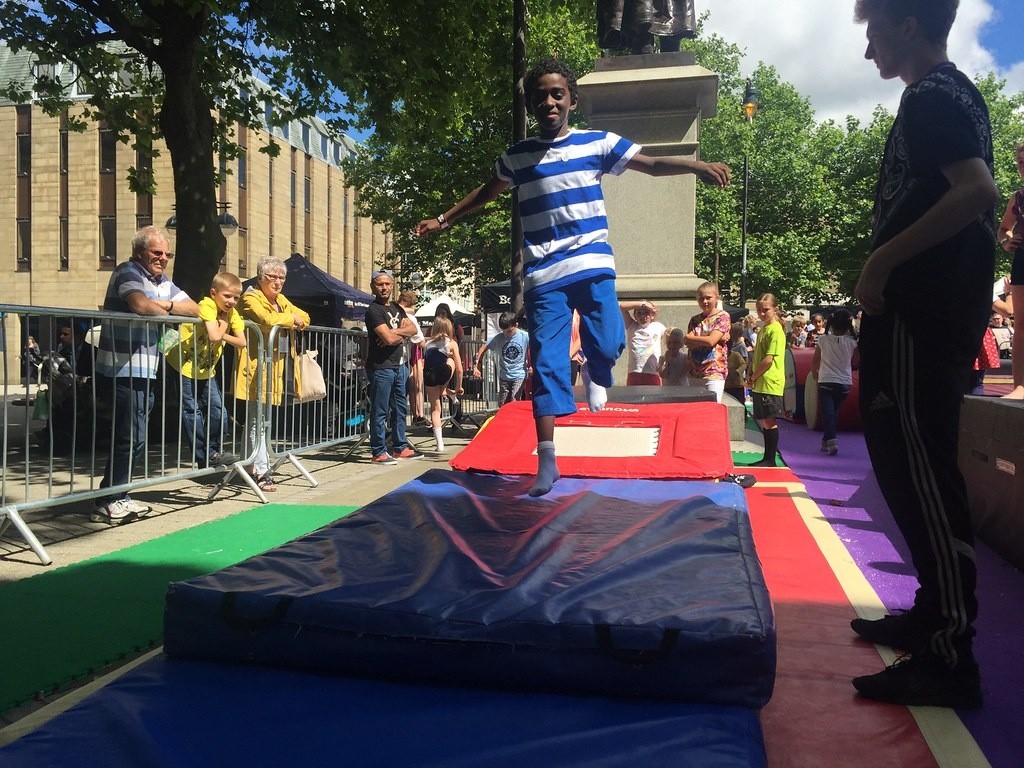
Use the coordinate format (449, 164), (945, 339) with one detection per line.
(417, 416), (431, 426)
(848, 605), (931, 651)
(826, 439), (839, 457)
(91, 503), (138, 525)
(854, 642), (980, 708)
(822, 439), (829, 452)
(393, 447), (425, 460)
(116, 495), (152, 517)
(370, 452), (397, 465)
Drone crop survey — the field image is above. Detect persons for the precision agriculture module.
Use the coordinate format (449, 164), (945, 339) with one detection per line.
(230, 255), (470, 492)
(849, 0), (996, 705)
(474, 312), (533, 407)
(89, 225), (201, 524)
(964, 276), (1013, 395)
(569, 282), (863, 467)
(997, 145), (1024, 389)
(596, 0), (698, 54)
(417, 61), (732, 498)
(167, 272), (247, 469)
(24, 322), (98, 441)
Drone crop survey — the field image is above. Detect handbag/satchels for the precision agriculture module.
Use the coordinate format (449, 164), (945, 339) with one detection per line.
(299, 347), (326, 403)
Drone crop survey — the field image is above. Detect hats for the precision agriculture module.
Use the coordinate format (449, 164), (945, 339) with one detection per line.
(371, 270), (394, 283)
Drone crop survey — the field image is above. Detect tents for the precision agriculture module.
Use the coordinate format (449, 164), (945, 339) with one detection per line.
(453, 310), (474, 328)
(481, 277), (525, 380)
(241, 253), (376, 322)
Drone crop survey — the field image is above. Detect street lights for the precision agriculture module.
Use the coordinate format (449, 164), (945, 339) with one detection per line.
(384, 255), (426, 295)
(739, 75), (759, 309)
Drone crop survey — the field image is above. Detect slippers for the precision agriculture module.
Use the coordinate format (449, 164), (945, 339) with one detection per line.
(257, 475), (276, 493)
(243, 473), (259, 486)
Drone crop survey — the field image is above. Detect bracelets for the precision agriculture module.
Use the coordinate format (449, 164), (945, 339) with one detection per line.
(1005, 292), (1012, 296)
(437, 214), (448, 230)
(166, 301), (173, 312)
(643, 304), (646, 308)
(1001, 238), (1008, 244)
(663, 362), (668, 366)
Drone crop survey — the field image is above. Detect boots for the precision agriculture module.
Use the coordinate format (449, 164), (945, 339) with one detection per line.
(748, 429), (778, 467)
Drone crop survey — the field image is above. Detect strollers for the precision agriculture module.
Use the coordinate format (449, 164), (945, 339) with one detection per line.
(323, 381), (391, 441)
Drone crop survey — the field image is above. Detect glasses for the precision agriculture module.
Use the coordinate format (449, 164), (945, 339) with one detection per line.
(141, 246), (174, 260)
(265, 274), (285, 282)
